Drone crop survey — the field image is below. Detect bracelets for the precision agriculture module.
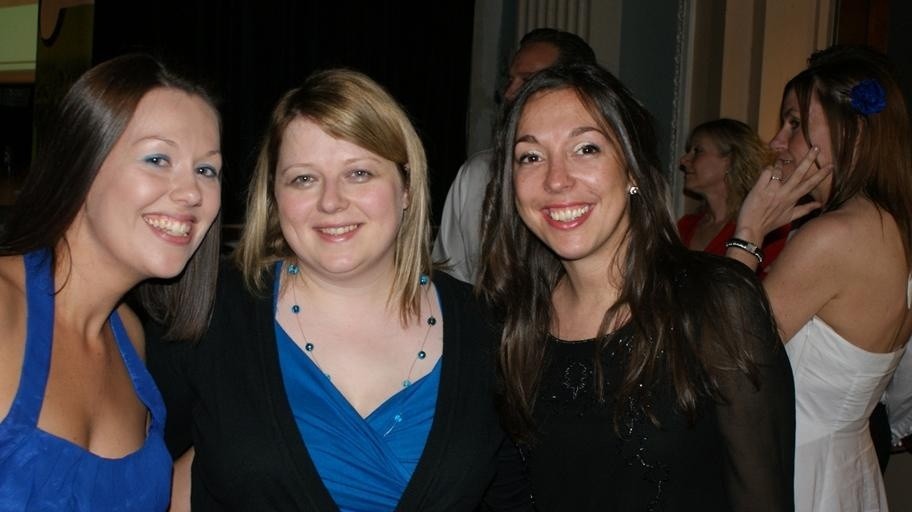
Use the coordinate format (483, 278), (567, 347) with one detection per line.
(724, 237), (765, 263)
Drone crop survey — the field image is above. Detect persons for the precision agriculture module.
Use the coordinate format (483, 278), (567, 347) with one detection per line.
(192, 68), (500, 511)
(0, 51), (224, 512)
(672, 119), (794, 281)
(483, 60), (795, 511)
(723, 38), (911, 512)
(430, 26), (595, 285)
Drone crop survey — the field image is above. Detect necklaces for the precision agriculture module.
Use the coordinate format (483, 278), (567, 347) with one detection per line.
(288, 258), (437, 440)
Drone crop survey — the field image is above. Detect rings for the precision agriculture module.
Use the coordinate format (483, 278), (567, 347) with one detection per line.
(770, 176), (783, 182)
